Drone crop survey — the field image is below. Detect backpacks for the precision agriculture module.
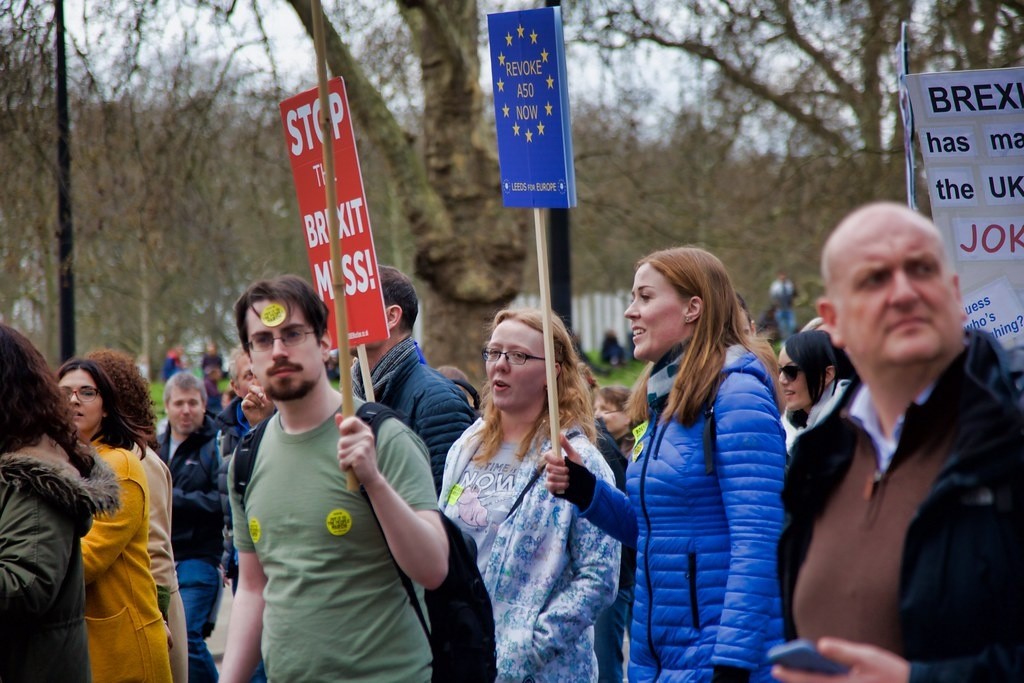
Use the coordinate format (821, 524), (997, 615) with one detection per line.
(233, 401), (498, 683)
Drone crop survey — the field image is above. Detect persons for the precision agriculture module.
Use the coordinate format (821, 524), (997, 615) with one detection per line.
(83, 350), (189, 683)
(544, 247), (788, 683)
(218, 274), (449, 683)
(156, 371), (223, 683)
(201, 342), (236, 413)
(161, 344), (184, 383)
(771, 270), (796, 334)
(780, 203), (1024, 683)
(56, 355), (173, 683)
(587, 384), (643, 683)
(216, 344), (277, 683)
(438, 308), (622, 683)
(778, 317), (856, 453)
(242, 263), (498, 683)
(0, 323), (120, 683)
(571, 329), (628, 377)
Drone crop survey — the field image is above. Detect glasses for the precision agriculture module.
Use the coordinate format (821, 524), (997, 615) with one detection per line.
(63, 386), (101, 403)
(479, 347), (564, 366)
(776, 364), (805, 383)
(247, 326), (318, 352)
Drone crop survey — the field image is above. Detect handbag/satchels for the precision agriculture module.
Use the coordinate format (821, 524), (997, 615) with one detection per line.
(157, 585), (170, 627)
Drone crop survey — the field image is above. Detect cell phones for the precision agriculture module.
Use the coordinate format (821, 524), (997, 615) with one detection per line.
(767, 638), (849, 674)
